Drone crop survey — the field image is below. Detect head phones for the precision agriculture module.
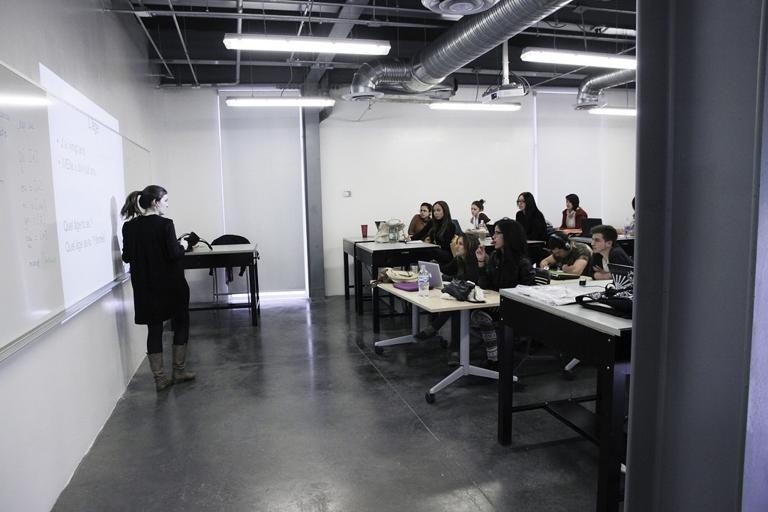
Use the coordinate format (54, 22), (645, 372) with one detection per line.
(544, 233), (571, 251)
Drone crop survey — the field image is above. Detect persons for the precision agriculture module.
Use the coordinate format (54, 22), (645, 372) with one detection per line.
(406, 189), (638, 370)
(119, 184), (197, 393)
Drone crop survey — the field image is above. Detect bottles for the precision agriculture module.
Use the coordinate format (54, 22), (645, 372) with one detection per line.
(579, 276), (585, 286)
(415, 264), (429, 297)
(623, 217), (630, 239)
(478, 223), (486, 240)
(388, 224), (397, 243)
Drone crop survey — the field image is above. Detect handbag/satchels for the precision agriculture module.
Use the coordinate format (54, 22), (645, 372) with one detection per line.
(441, 278), (475, 301)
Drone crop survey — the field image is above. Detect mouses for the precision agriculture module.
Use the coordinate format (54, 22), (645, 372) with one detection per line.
(580, 278), (586, 285)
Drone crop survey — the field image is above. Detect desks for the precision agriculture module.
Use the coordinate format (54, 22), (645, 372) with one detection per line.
(170, 243), (262, 327)
(342, 227), (636, 508)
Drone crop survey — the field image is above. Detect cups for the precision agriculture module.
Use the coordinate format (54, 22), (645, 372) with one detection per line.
(361, 224), (367, 238)
(374, 221), (384, 230)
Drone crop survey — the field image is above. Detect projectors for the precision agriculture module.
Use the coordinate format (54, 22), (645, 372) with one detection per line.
(482, 84), (525, 104)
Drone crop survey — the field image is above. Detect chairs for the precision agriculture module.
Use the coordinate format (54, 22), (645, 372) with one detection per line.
(212, 234), (253, 314)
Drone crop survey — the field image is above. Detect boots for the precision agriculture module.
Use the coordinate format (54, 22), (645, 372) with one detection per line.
(147, 353), (173, 392)
(172, 343), (196, 383)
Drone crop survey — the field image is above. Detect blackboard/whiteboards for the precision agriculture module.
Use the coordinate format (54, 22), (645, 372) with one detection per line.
(0, 60), (152, 362)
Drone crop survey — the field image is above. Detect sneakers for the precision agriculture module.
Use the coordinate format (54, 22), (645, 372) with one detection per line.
(414, 331), (436, 341)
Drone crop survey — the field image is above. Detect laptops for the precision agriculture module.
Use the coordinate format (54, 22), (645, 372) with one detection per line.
(581, 219), (602, 237)
(418, 260), (449, 289)
(608, 263), (633, 282)
(613, 239), (634, 262)
(452, 220), (475, 233)
(549, 271), (580, 280)
(486, 224), (496, 241)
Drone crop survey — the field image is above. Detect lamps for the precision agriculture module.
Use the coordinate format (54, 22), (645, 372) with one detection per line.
(518, 27), (635, 73)
(226, 64), (337, 110)
(427, 75), (524, 112)
(587, 83), (637, 118)
(224, 2), (393, 58)
